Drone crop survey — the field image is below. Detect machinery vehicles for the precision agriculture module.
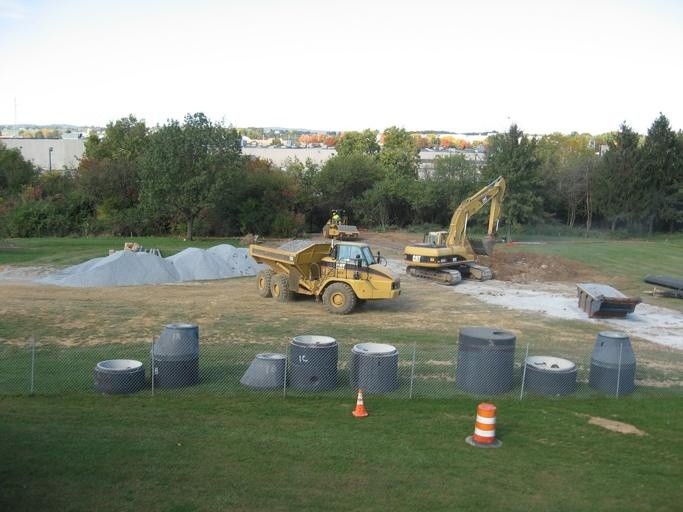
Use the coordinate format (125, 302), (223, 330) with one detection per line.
(403, 176), (506, 286)
(322, 210), (360, 241)
(249, 239), (401, 316)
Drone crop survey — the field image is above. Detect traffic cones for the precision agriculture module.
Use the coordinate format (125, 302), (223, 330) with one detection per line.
(352, 389), (368, 417)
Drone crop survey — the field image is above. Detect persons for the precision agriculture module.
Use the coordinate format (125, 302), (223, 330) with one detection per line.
(331, 211), (341, 223)
